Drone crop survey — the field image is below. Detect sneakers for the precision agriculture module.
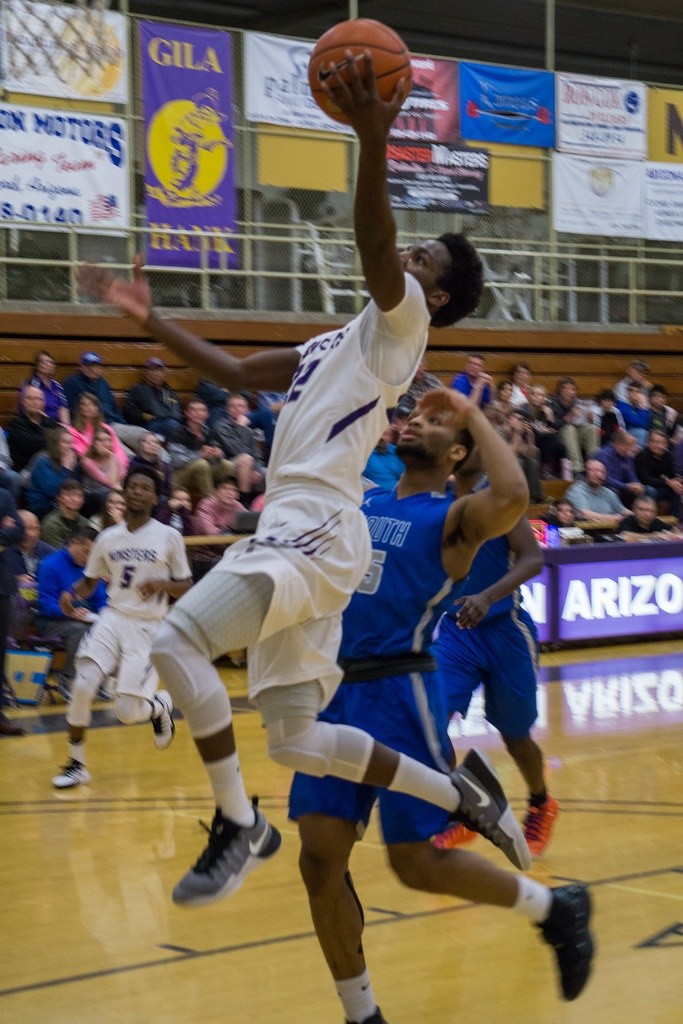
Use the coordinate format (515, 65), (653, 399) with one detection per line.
(172, 795), (281, 906)
(150, 689), (176, 751)
(536, 884), (593, 1001)
(450, 748), (530, 871)
(51, 755), (91, 787)
(432, 823), (476, 850)
(524, 794), (560, 857)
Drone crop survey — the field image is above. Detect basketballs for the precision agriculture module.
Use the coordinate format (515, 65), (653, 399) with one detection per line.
(307, 16), (415, 127)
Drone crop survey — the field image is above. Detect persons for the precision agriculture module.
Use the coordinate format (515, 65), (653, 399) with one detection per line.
(423, 440), (555, 856)
(0, 351), (683, 696)
(50, 466), (195, 789)
(75, 48), (532, 901)
(289, 388), (594, 1024)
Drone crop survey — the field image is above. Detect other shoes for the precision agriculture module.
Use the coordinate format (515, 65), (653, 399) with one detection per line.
(55, 668), (76, 704)
(0, 712), (25, 735)
(96, 686), (113, 701)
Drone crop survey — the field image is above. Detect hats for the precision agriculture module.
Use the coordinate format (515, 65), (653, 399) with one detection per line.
(514, 408), (536, 423)
(146, 356), (165, 370)
(80, 352), (101, 365)
